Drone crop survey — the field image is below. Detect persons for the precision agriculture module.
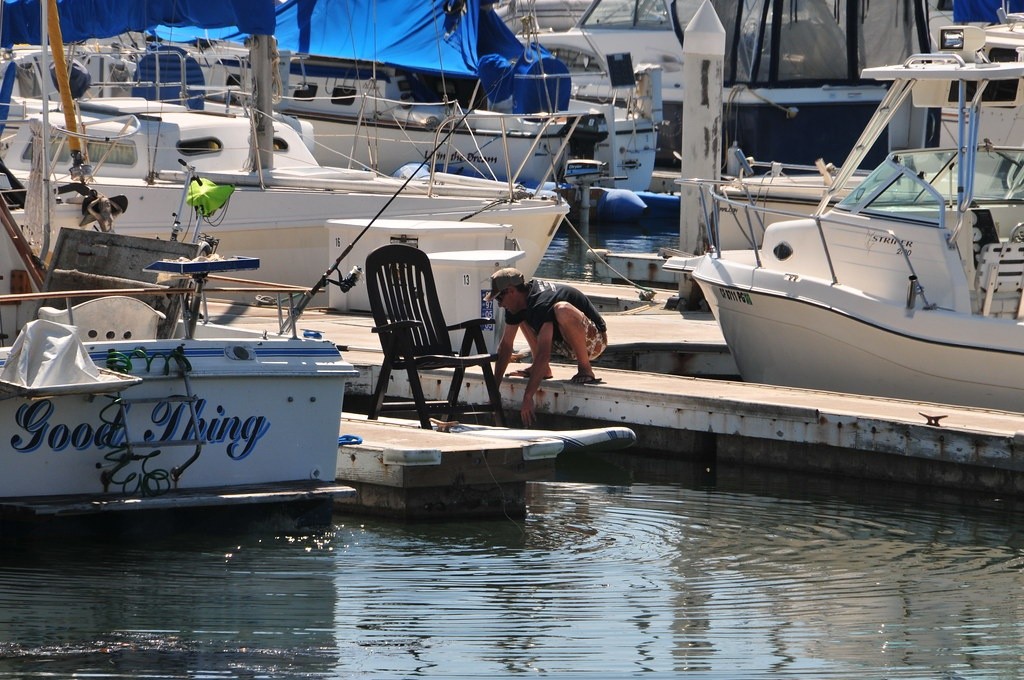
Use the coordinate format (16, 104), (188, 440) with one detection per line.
(483, 267), (608, 428)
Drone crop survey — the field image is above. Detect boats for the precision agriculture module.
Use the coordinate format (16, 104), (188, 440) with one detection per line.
(0, 171), (366, 518)
(689, 23), (1024, 412)
(1, 0), (1019, 334)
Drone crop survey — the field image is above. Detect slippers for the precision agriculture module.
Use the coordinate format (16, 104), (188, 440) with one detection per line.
(519, 370), (553, 379)
(570, 373), (602, 384)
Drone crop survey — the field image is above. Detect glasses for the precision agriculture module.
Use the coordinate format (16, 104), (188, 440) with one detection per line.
(495, 288), (509, 303)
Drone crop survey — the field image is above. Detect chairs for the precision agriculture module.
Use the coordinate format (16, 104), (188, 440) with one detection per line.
(365, 244), (508, 430)
(38, 295), (166, 342)
(974, 241), (1024, 319)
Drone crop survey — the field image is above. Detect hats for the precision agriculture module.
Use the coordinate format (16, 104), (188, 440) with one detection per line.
(483, 267), (524, 301)
